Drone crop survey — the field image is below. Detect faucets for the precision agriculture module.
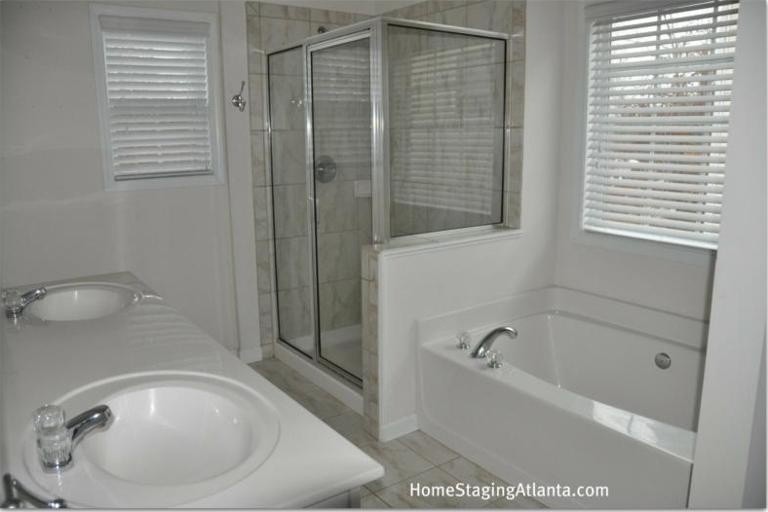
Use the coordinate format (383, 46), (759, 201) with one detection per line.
(2, 286), (47, 320)
(473, 324), (520, 359)
(31, 403), (115, 475)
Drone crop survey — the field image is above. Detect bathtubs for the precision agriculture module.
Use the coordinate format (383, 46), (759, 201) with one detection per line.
(417, 308), (709, 509)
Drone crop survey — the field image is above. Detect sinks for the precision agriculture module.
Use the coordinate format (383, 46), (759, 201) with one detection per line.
(22, 280), (145, 322)
(20, 370), (281, 510)
